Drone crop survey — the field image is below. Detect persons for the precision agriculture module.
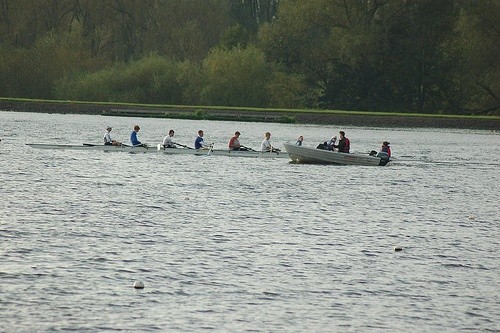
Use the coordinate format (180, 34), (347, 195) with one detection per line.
(229, 131), (255, 151)
(130, 126), (147, 147)
(261, 132), (282, 155)
(382, 141), (391, 157)
(195, 130), (214, 150)
(327, 131), (350, 153)
(296, 136), (304, 146)
(103, 125), (122, 146)
(162, 130), (176, 148)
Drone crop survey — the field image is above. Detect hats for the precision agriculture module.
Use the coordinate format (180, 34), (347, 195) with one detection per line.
(106, 125), (113, 129)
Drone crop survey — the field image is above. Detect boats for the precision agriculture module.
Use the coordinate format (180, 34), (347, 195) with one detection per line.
(282, 142), (381, 166)
(25, 142), (287, 158)
